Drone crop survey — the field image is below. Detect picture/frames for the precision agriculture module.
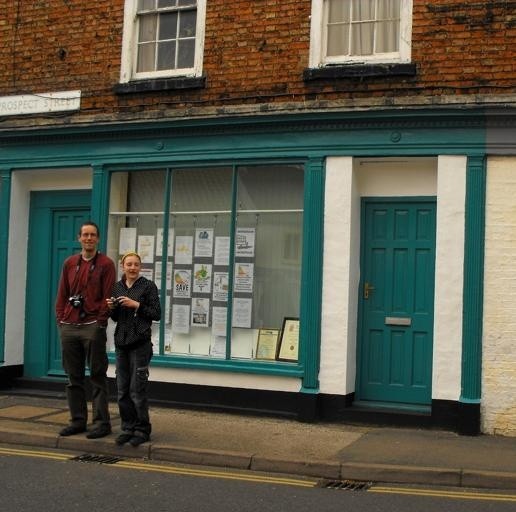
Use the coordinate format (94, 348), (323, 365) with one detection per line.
(255, 329), (279, 361)
(274, 316), (299, 362)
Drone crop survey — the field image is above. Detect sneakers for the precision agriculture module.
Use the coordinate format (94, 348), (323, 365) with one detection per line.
(87, 430), (110, 439)
(58, 425), (87, 436)
(116, 434), (150, 445)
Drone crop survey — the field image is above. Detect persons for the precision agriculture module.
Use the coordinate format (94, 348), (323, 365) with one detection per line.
(55, 222), (116, 439)
(106, 251), (161, 446)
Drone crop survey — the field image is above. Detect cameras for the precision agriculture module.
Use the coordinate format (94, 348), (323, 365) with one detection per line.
(69, 295), (84, 309)
(111, 298), (123, 306)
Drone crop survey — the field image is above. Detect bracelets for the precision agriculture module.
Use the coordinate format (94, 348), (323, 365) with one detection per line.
(134, 302), (139, 318)
